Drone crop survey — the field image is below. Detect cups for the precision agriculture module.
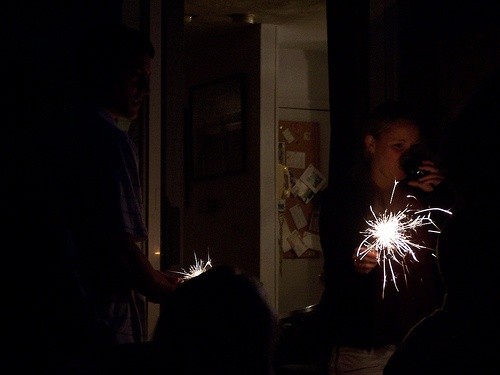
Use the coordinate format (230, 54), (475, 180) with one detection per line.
(399, 148), (433, 179)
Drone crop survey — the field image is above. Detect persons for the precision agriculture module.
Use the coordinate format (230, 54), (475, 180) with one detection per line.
(317, 100), (446, 374)
(34, 22), (183, 375)
